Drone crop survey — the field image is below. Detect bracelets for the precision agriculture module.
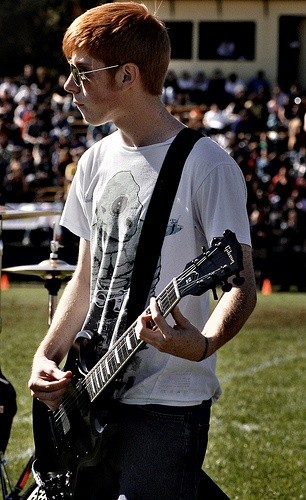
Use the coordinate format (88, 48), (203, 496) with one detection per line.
(197, 337), (210, 364)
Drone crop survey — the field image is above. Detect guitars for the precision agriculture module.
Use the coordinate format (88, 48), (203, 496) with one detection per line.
(30, 229), (246, 500)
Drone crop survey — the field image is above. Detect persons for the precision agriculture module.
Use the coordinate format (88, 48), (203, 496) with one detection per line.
(29, 2), (257, 500)
(0, 62), (305, 297)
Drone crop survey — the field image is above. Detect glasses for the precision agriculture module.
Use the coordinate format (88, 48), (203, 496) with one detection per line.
(68, 60), (141, 86)
